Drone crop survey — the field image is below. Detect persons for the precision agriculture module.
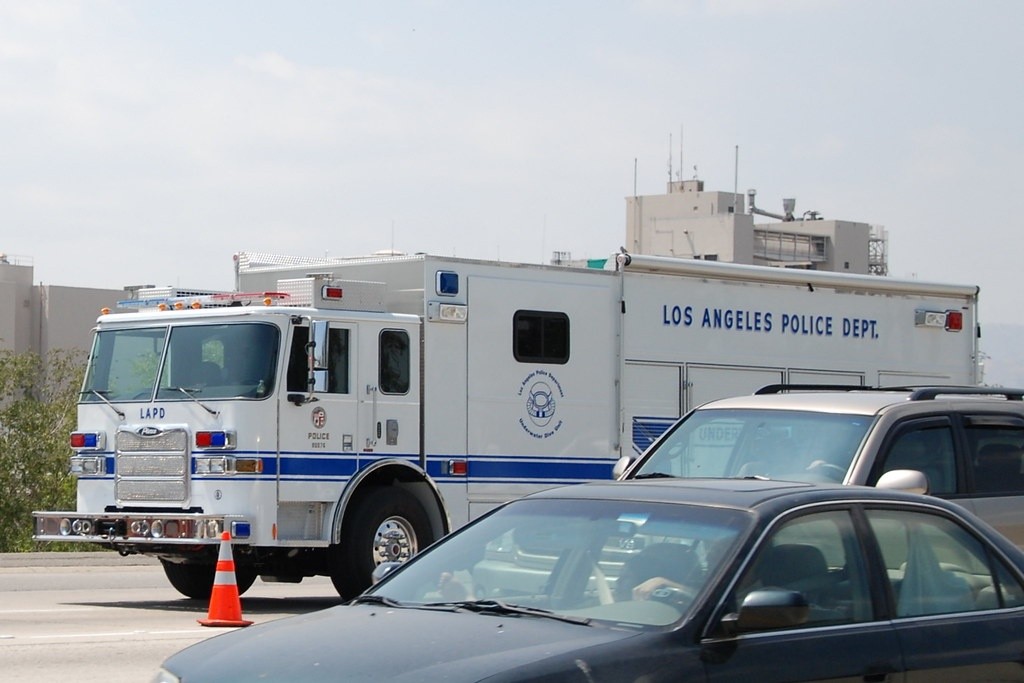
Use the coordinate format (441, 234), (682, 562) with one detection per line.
(632, 536), (768, 616)
(806, 428), (944, 494)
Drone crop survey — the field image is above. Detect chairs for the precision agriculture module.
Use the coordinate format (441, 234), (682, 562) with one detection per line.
(974, 443), (1024, 494)
(616, 541), (703, 601)
(767, 545), (849, 624)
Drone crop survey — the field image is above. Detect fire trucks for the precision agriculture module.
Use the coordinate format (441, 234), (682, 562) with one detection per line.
(30, 243), (988, 604)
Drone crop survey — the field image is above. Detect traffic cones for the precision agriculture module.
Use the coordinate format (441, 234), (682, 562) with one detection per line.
(195, 532), (254, 626)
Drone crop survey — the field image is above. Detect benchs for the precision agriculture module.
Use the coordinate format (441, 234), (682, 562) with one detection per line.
(886, 561), (1008, 612)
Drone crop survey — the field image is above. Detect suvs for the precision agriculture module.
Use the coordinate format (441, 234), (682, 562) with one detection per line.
(476, 386), (1024, 581)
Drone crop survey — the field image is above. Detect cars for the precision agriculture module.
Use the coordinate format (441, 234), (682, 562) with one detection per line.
(148, 478), (1024, 683)
